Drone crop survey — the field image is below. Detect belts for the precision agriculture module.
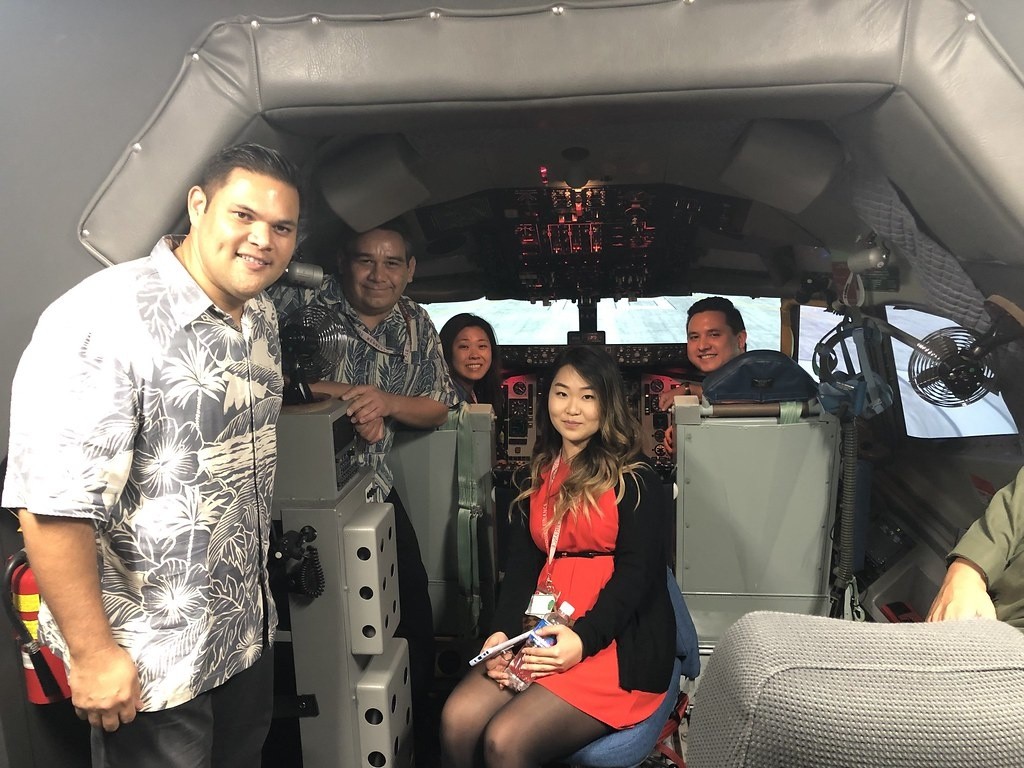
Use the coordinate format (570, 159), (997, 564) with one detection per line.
(547, 550), (615, 558)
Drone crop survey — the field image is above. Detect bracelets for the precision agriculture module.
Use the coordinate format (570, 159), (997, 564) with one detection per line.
(680, 382), (691, 394)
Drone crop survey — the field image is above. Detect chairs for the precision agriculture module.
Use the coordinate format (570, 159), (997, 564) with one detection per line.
(684, 610), (1024, 768)
(658, 351), (844, 655)
(387, 402), (505, 644)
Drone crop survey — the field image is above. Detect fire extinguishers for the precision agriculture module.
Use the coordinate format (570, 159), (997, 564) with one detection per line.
(2, 546), (72, 707)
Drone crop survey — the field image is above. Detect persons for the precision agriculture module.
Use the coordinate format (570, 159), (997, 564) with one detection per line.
(925, 465), (1023, 630)
(272, 227), (459, 709)
(0, 141), (299, 768)
(438, 312), (503, 402)
(659, 295), (749, 454)
(440, 346), (677, 768)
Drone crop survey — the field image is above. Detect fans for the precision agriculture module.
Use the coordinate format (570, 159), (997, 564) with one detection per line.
(907, 294), (1024, 408)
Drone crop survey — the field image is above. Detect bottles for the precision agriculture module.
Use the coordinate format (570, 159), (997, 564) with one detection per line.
(501, 601), (575, 692)
